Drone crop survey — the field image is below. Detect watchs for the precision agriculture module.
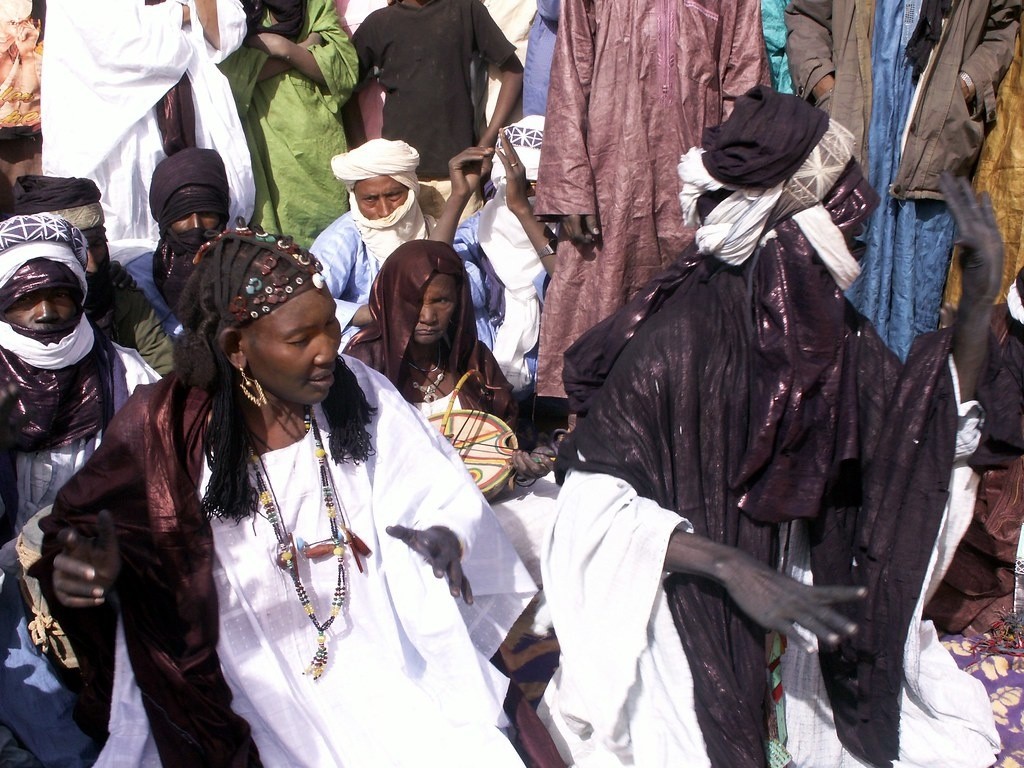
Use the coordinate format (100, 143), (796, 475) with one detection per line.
(537, 238), (558, 258)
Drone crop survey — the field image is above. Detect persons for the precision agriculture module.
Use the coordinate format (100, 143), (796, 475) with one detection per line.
(0, 0), (1024, 478)
(37, 231), (568, 768)
(545, 83), (1005, 768)
(0, 212), (163, 544)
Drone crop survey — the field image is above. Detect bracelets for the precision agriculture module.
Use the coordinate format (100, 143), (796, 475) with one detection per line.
(959, 72), (975, 104)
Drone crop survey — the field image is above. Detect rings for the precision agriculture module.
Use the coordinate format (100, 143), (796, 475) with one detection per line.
(511, 162), (518, 167)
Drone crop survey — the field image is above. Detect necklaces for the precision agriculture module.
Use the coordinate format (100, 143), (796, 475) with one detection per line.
(409, 341), (441, 372)
(412, 359), (446, 403)
(249, 405), (345, 680)
